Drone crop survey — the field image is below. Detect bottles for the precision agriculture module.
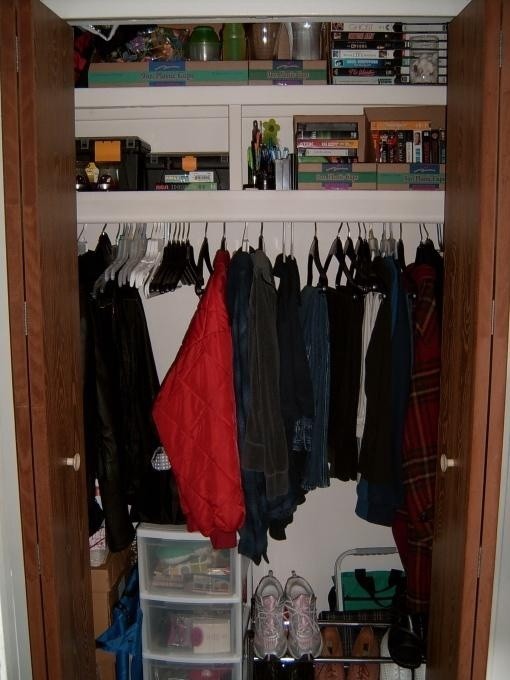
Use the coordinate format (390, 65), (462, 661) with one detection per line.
(410, 34), (440, 86)
(188, 26), (220, 60)
(221, 25), (245, 60)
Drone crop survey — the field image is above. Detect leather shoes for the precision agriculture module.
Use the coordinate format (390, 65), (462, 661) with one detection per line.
(315, 613), (427, 680)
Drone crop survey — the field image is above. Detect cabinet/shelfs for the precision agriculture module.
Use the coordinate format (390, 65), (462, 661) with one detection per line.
(74, 85), (447, 223)
(136, 522), (253, 680)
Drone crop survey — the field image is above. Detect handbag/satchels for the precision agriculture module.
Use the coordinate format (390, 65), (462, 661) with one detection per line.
(96, 565), (142, 680)
(328, 568), (404, 611)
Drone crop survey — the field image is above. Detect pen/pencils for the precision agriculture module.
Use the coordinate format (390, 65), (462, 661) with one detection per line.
(248, 118), (288, 170)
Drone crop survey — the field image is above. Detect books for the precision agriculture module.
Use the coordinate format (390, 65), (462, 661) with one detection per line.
(296, 121), (446, 191)
(330, 22), (447, 85)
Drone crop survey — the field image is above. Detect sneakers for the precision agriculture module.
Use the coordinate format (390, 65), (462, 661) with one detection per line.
(285, 571), (322, 660)
(252, 570), (288, 661)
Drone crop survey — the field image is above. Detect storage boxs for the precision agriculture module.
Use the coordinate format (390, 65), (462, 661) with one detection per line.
(90, 549), (129, 680)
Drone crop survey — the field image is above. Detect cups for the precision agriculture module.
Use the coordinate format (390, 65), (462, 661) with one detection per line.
(291, 21), (321, 62)
(246, 22), (282, 60)
